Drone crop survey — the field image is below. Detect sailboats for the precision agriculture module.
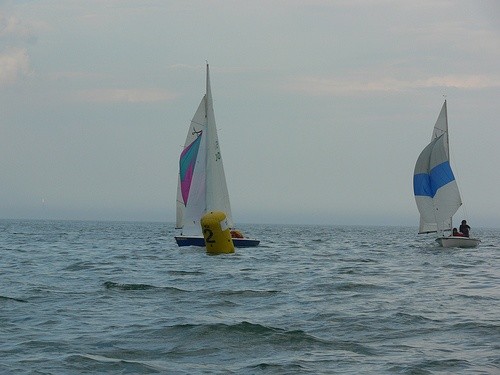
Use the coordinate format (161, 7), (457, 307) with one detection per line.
(173, 63), (261, 248)
(412, 99), (482, 249)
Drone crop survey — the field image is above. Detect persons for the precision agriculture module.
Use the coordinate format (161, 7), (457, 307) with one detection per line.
(460, 220), (470, 237)
(453, 228), (459, 234)
